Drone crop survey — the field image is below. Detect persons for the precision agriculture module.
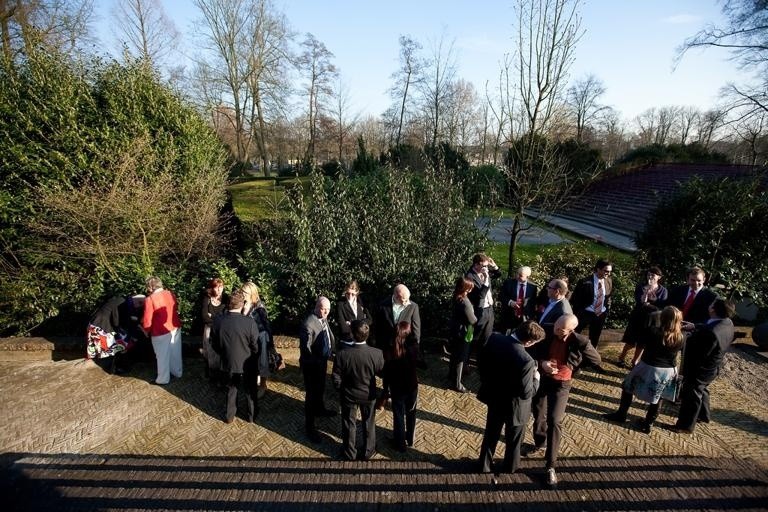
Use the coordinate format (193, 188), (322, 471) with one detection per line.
(379, 320), (420, 453)
(528, 312), (602, 492)
(567, 259), (614, 372)
(137, 276), (184, 386)
(200, 276), (285, 424)
(374, 281), (422, 416)
(330, 318), (386, 462)
(600, 306), (687, 434)
(336, 279), (373, 350)
(647, 266), (720, 406)
(537, 279), (575, 327)
(617, 266), (669, 371)
(499, 266), (540, 330)
(299, 296), (337, 443)
(535, 274), (571, 323)
(668, 297), (735, 434)
(445, 276), (478, 392)
(467, 254), (501, 370)
(85, 293), (145, 375)
(473, 320), (546, 474)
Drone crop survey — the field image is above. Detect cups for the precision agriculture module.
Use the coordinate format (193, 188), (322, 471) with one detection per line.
(550, 359), (558, 374)
(531, 359), (540, 376)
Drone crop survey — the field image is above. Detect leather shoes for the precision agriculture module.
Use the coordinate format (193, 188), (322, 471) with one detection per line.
(667, 424), (693, 434)
(309, 429), (323, 444)
(700, 416), (710, 424)
(451, 383), (471, 393)
(316, 408), (337, 417)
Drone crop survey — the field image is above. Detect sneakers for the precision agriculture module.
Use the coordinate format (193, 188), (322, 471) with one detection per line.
(389, 439), (413, 454)
(545, 466), (558, 485)
(525, 447), (546, 461)
(639, 417), (654, 435)
(377, 390), (389, 410)
(603, 411), (627, 424)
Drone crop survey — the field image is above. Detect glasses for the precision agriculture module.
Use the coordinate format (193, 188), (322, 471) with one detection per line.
(547, 285), (558, 289)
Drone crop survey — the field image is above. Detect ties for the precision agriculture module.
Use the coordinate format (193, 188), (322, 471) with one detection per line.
(594, 282), (603, 318)
(321, 318), (332, 358)
(517, 283), (526, 318)
(682, 289), (696, 320)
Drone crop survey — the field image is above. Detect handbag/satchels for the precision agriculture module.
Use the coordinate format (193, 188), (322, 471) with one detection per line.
(637, 310), (663, 348)
(267, 348), (286, 372)
